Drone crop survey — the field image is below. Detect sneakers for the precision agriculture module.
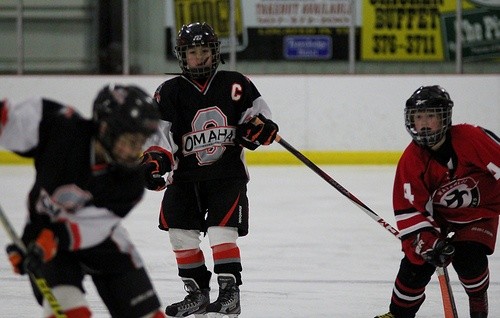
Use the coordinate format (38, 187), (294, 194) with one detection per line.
(205, 273), (241, 318)
(164, 271), (213, 318)
(469, 289), (488, 318)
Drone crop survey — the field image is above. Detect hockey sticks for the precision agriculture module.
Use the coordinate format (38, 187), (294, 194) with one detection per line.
(436, 258), (457, 318)
(0, 210), (65, 318)
(252, 116), (400, 238)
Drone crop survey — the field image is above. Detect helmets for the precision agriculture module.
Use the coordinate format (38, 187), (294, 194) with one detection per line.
(175, 22), (221, 81)
(404, 85), (455, 147)
(92, 83), (161, 174)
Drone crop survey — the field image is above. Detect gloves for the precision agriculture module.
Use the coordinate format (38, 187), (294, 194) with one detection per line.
(412, 232), (456, 267)
(6, 222), (69, 276)
(136, 150), (173, 191)
(242, 113), (279, 145)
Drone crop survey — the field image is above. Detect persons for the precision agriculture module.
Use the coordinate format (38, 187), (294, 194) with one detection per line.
(374, 85), (500, 318)
(0, 84), (166, 318)
(138, 21), (278, 318)
